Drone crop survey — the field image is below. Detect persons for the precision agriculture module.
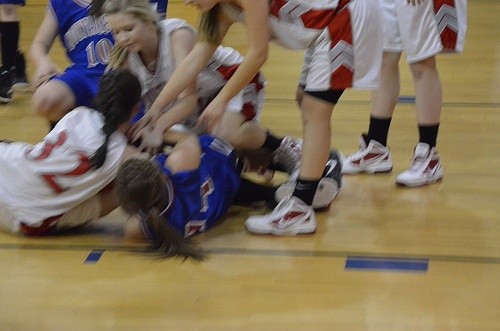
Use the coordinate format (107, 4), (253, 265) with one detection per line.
(0, 0), (383, 263)
(341, 0), (469, 187)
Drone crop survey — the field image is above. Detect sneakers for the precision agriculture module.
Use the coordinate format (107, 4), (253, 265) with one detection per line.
(273, 139), (303, 183)
(245, 198), (316, 236)
(396, 147), (443, 188)
(339, 134), (393, 175)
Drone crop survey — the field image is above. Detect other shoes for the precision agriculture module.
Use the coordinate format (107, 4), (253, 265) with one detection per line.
(10, 56), (29, 89)
(275, 179), (338, 208)
(0, 67), (11, 102)
